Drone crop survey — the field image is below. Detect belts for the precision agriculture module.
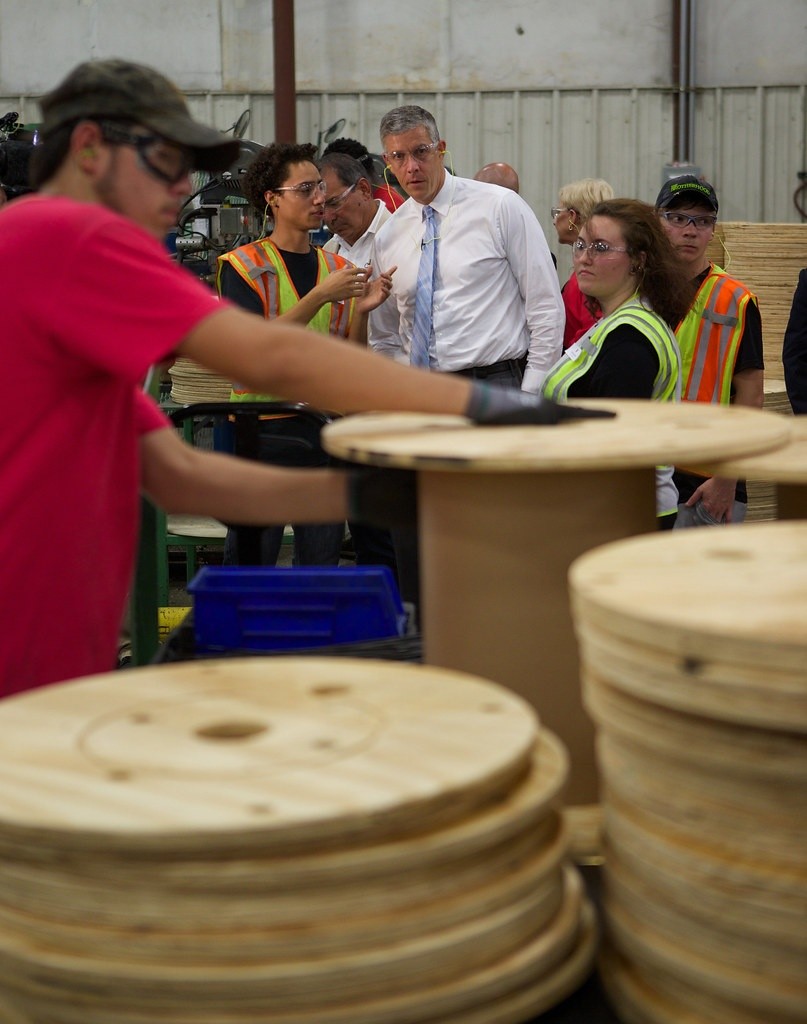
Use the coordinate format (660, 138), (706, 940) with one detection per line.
(446, 357), (526, 381)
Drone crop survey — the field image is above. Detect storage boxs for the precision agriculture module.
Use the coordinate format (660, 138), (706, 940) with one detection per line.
(186, 564), (405, 657)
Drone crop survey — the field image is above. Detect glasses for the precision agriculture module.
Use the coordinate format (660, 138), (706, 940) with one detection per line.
(658, 208), (719, 230)
(322, 183), (357, 213)
(547, 205), (565, 220)
(98, 125), (198, 184)
(268, 182), (327, 198)
(382, 140), (440, 163)
(572, 239), (633, 261)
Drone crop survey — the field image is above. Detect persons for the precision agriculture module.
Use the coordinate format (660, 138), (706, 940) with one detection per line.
(648, 170), (767, 531)
(214, 141), (398, 568)
(0, 55), (621, 710)
(549, 178), (616, 356)
(322, 135), (406, 214)
(540, 195), (700, 533)
(318, 151), (397, 274)
(474, 162), (519, 194)
(363, 104), (569, 395)
(783, 269), (807, 418)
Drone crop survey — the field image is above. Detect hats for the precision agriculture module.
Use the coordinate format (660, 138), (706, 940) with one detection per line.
(654, 176), (720, 214)
(36, 57), (242, 174)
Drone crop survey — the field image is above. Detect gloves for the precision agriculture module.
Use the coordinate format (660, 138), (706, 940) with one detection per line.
(464, 379), (617, 425)
(345, 463), (419, 536)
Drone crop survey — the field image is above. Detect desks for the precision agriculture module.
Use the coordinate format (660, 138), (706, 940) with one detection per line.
(153, 396), (357, 609)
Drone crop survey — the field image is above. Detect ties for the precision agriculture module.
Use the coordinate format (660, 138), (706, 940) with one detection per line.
(408, 207), (436, 370)
